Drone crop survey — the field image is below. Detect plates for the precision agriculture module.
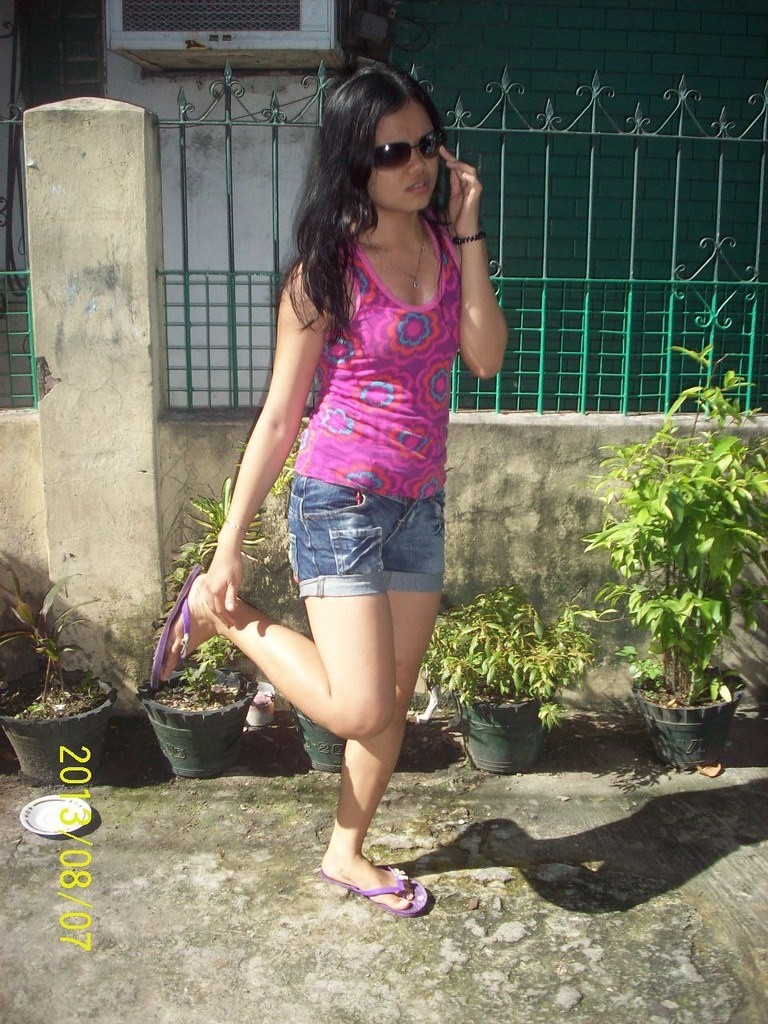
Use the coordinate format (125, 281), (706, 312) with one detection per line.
(21, 794), (92, 835)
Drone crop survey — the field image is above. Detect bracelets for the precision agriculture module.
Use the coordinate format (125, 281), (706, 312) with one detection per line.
(451, 230), (486, 244)
(224, 519), (246, 532)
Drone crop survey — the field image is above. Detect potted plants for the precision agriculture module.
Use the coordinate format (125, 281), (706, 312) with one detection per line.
(421, 343), (768, 775)
(0, 417), (405, 783)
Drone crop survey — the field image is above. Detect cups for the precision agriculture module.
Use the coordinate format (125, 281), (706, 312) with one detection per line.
(246, 683), (275, 726)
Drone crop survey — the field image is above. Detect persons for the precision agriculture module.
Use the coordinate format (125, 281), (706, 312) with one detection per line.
(150, 64), (508, 913)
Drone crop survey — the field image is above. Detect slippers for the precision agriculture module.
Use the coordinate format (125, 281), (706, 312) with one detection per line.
(321, 866), (428, 917)
(150, 564), (203, 690)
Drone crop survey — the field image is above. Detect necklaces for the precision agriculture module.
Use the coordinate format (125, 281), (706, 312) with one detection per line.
(366, 220), (428, 289)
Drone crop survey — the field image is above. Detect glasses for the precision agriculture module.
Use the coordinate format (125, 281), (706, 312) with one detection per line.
(373, 129), (443, 169)
